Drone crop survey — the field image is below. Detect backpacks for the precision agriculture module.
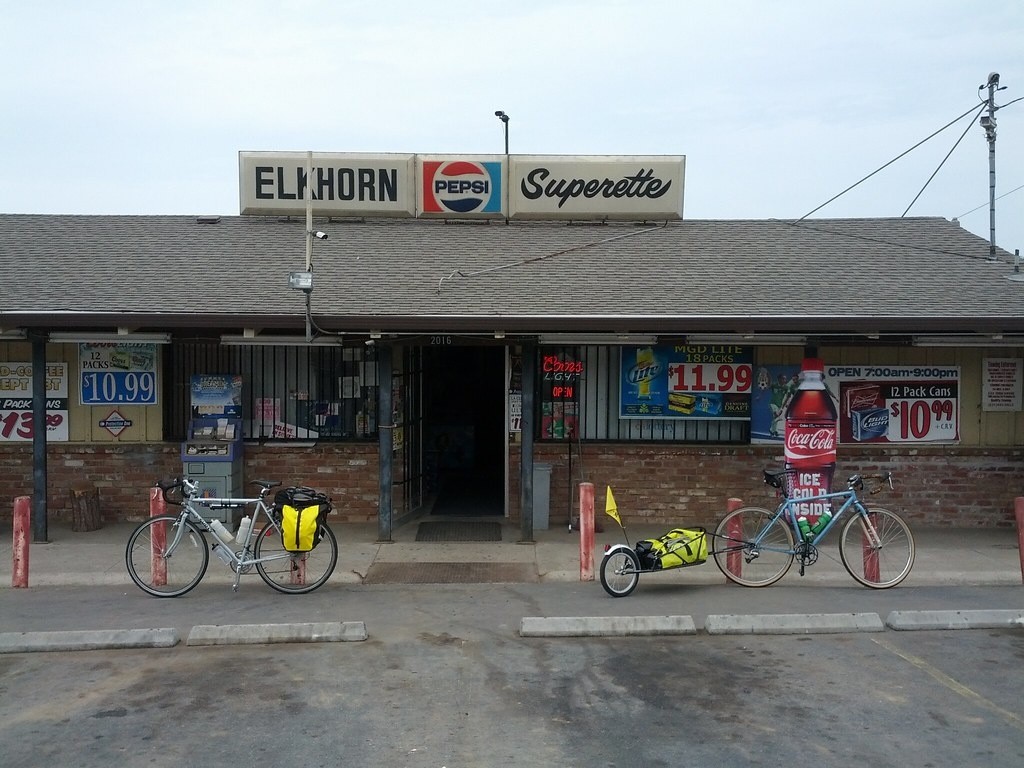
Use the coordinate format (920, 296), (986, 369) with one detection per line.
(273, 486), (333, 553)
(635, 528), (708, 570)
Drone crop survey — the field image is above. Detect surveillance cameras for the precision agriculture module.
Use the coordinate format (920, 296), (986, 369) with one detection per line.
(311, 229), (328, 239)
(980, 116), (997, 130)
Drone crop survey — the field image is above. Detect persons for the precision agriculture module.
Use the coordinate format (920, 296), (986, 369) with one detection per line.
(767, 371), (839, 436)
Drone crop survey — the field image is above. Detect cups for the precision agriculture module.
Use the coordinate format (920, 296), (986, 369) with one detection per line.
(636, 348), (655, 400)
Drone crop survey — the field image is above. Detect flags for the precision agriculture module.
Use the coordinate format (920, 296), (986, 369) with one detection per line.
(605, 486), (627, 528)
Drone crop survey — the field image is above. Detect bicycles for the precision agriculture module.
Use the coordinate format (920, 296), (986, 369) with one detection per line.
(713, 469), (915, 589)
(125, 476), (339, 599)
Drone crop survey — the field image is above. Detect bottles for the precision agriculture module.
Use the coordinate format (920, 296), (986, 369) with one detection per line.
(784, 358), (838, 526)
(234, 515), (251, 545)
(811, 506), (834, 534)
(210, 518), (234, 544)
(798, 517), (813, 542)
